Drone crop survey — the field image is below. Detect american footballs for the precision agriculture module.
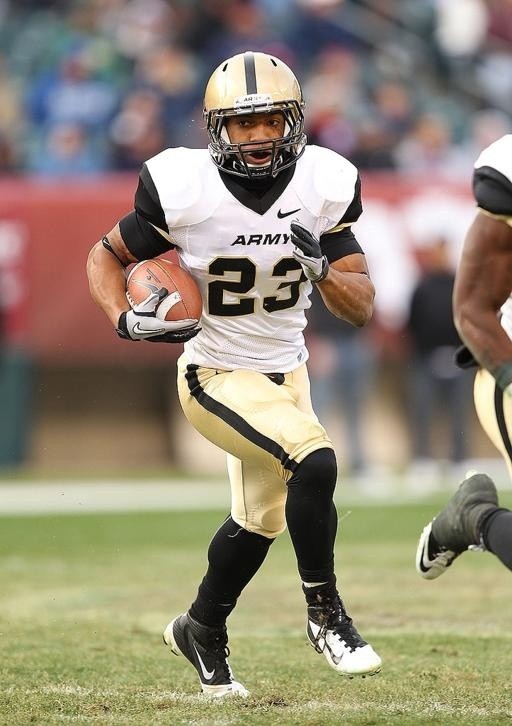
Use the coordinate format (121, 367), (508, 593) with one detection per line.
(125, 257), (203, 322)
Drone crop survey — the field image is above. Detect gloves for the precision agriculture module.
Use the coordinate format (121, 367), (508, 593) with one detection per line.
(288, 220), (333, 286)
(114, 287), (204, 343)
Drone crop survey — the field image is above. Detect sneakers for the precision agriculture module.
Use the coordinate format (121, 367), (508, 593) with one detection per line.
(413, 469), (500, 580)
(304, 597), (385, 679)
(162, 614), (252, 700)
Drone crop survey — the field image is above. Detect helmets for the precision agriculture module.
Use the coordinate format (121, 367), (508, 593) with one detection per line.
(202, 50), (306, 124)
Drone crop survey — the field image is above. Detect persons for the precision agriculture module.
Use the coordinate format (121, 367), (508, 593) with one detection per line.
(0, 0), (511, 475)
(85, 48), (380, 701)
(410, 136), (512, 583)
